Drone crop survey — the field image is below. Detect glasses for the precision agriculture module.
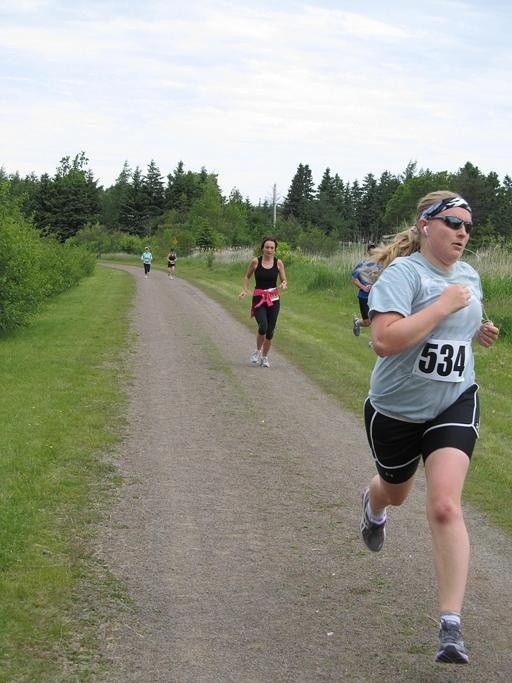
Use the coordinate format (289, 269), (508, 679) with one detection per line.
(427, 215), (473, 234)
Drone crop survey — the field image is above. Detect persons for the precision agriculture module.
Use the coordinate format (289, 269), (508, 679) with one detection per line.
(351, 245), (385, 336)
(360, 191), (499, 665)
(239, 237), (288, 368)
(167, 249), (177, 280)
(142, 247), (152, 279)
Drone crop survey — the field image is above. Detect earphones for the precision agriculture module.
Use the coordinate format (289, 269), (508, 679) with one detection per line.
(423, 226), (428, 237)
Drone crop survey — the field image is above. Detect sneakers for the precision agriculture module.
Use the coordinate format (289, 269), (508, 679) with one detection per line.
(360, 487), (387, 552)
(250, 349), (262, 365)
(144, 271), (150, 279)
(168, 273), (176, 281)
(262, 356), (270, 368)
(352, 316), (360, 336)
(435, 620), (470, 664)
